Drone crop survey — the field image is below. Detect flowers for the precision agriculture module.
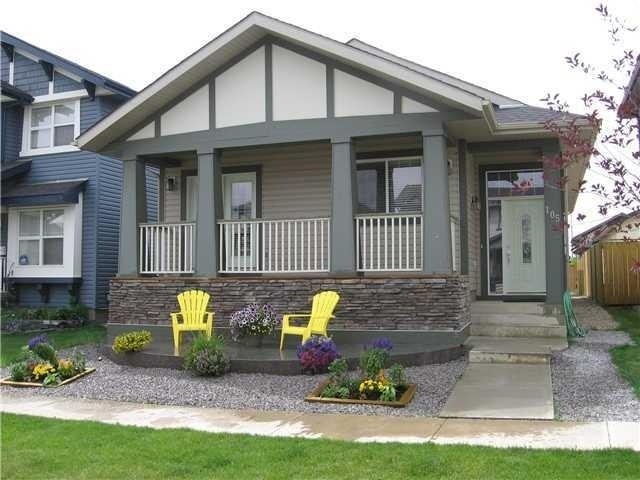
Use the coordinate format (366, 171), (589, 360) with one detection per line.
(182, 332), (232, 376)
(296, 335), (339, 375)
(112, 329), (152, 354)
(229, 304), (279, 343)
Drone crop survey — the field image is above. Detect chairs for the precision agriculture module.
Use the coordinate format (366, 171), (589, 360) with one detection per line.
(170, 289), (216, 357)
(279, 289), (340, 350)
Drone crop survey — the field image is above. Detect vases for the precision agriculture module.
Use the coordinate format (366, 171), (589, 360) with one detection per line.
(241, 336), (265, 348)
